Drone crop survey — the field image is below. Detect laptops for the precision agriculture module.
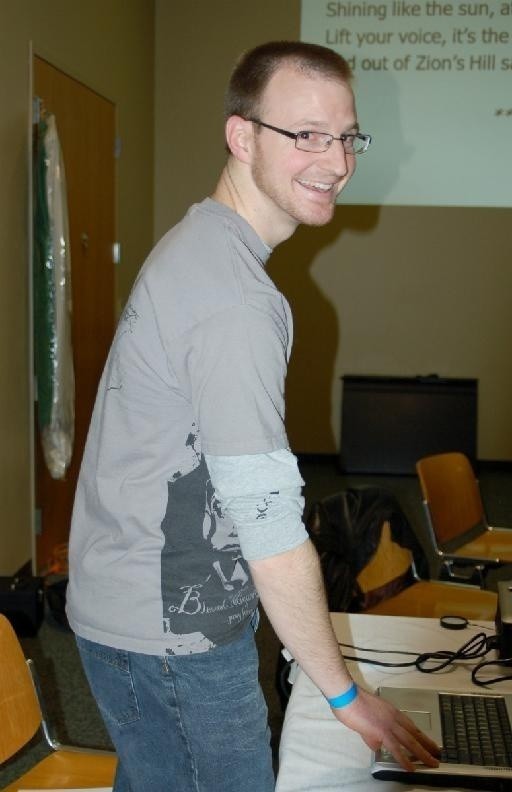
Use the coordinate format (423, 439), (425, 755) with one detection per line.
(372, 686), (512, 792)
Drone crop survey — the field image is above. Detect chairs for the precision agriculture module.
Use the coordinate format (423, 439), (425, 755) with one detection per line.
(0, 612), (119, 792)
(306, 453), (512, 621)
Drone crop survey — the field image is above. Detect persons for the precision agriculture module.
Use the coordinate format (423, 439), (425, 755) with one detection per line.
(52, 38), (448, 790)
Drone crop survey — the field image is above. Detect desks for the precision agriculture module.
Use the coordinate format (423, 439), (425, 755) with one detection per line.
(276, 612), (512, 792)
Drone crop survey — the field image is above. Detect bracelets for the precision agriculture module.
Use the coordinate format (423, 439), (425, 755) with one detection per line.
(324, 685), (360, 711)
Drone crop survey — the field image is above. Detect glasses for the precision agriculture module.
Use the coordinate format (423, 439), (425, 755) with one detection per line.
(245, 115), (372, 157)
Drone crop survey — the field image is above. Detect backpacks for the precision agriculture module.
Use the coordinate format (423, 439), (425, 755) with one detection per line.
(273, 485), (431, 715)
